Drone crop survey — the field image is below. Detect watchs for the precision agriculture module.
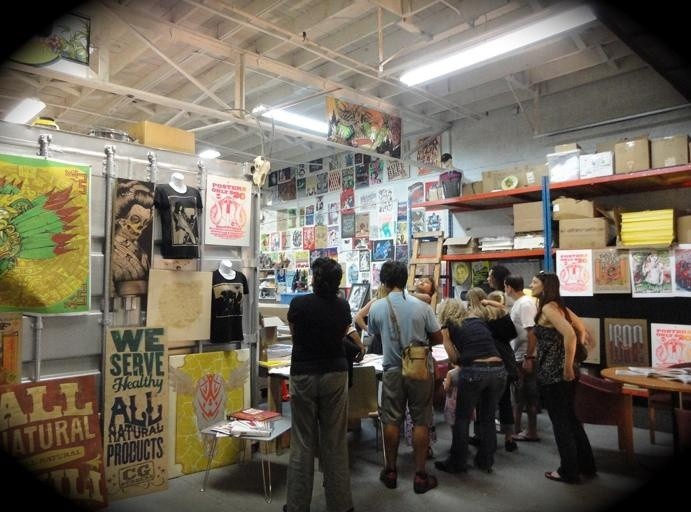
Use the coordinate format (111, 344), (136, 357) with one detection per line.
(523, 353), (536, 360)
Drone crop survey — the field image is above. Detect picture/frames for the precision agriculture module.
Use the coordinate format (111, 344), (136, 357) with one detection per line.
(602, 316), (650, 368)
(347, 283), (370, 312)
(650, 322), (691, 368)
(576, 315), (602, 365)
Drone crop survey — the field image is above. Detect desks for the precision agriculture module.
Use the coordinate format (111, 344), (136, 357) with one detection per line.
(600, 365), (691, 459)
(200, 418), (291, 504)
(269, 343), (449, 471)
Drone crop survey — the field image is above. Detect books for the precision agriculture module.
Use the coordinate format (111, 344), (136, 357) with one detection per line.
(210, 406), (281, 439)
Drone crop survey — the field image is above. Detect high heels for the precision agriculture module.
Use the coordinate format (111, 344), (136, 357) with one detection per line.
(474, 453), (496, 474)
(434, 459), (467, 474)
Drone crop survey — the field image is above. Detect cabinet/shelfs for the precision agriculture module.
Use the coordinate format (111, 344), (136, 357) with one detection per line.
(405, 176), (546, 302)
(546, 162), (691, 433)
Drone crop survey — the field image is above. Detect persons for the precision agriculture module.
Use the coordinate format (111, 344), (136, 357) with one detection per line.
(480, 290), (507, 307)
(154, 172), (204, 260)
(442, 360), (477, 455)
(353, 284), (389, 418)
(439, 152), (464, 199)
(467, 287), (519, 452)
(503, 273), (543, 442)
(282, 257), (366, 512)
(210, 258), (249, 345)
(486, 264), (516, 435)
(405, 275), (438, 459)
(434, 296), (507, 475)
(367, 260), (444, 495)
(172, 201), (197, 245)
(109, 180), (154, 297)
(530, 273), (599, 485)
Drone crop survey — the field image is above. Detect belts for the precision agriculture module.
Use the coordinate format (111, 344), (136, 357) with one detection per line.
(466, 359), (508, 369)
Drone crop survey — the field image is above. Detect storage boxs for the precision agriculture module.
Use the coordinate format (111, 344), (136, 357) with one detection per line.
(615, 138), (650, 175)
(443, 236), (479, 254)
(559, 217), (610, 249)
(651, 134), (688, 170)
(512, 202), (544, 234)
(552, 194), (599, 221)
(546, 149), (579, 184)
(129, 120), (195, 155)
(579, 150), (614, 179)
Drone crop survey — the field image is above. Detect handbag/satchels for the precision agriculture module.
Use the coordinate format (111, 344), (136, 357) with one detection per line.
(385, 297), (433, 381)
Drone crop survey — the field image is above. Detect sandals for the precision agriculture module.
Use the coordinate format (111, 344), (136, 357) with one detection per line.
(543, 470), (585, 484)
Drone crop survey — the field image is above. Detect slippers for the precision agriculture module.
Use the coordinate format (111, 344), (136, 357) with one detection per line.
(379, 468), (399, 489)
(412, 472), (440, 493)
(512, 431), (542, 443)
